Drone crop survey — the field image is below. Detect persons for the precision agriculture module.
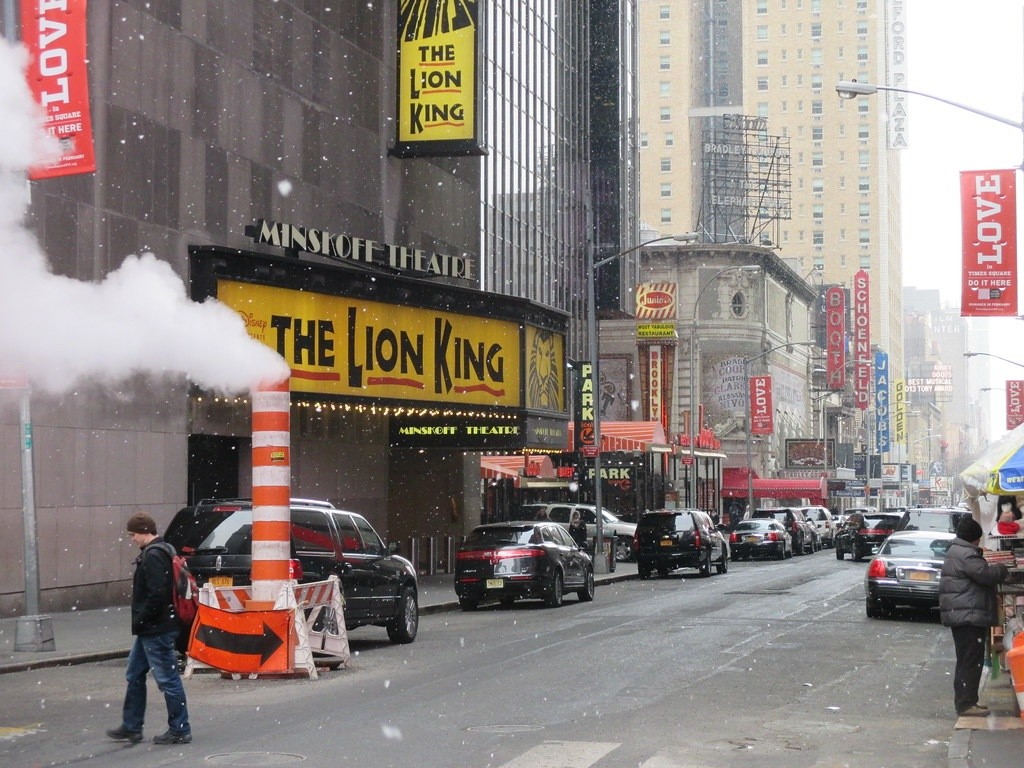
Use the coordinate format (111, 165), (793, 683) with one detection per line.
(536, 508), (588, 553)
(939, 517), (1007, 717)
(106, 513), (192, 746)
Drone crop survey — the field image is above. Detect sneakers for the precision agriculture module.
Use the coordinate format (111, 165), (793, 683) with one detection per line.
(106, 724), (144, 742)
(153, 729), (192, 744)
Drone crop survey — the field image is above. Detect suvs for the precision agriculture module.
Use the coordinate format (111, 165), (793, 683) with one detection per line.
(752, 508), (814, 557)
(163, 497), (419, 644)
(638, 508), (728, 579)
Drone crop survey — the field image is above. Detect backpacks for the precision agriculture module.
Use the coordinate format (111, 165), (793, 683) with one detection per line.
(138, 541), (199, 624)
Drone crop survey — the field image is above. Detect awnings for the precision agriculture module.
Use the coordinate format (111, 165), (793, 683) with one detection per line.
(721, 477), (827, 499)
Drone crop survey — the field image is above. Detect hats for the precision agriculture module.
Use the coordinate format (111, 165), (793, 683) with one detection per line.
(956, 516), (982, 542)
(127, 511), (156, 533)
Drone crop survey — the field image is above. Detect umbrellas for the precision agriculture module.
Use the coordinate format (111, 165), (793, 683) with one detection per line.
(959, 421), (1024, 500)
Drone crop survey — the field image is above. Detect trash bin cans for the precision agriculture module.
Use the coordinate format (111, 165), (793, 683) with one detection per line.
(592, 535), (619, 572)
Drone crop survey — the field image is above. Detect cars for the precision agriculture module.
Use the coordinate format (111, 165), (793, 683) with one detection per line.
(834, 512), (903, 562)
(798, 506), (910, 549)
(729, 519), (793, 561)
(806, 518), (822, 552)
(864, 531), (957, 617)
(454, 521), (594, 612)
(894, 508), (972, 533)
(524, 504), (637, 562)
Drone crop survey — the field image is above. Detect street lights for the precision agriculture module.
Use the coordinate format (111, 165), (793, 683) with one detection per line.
(691, 264), (762, 508)
(879, 411), (943, 507)
(743, 340), (817, 519)
(825, 360), (872, 473)
(587, 233), (698, 574)
(867, 401), (912, 476)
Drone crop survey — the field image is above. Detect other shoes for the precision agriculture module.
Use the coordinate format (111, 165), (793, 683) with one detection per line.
(958, 703), (991, 717)
